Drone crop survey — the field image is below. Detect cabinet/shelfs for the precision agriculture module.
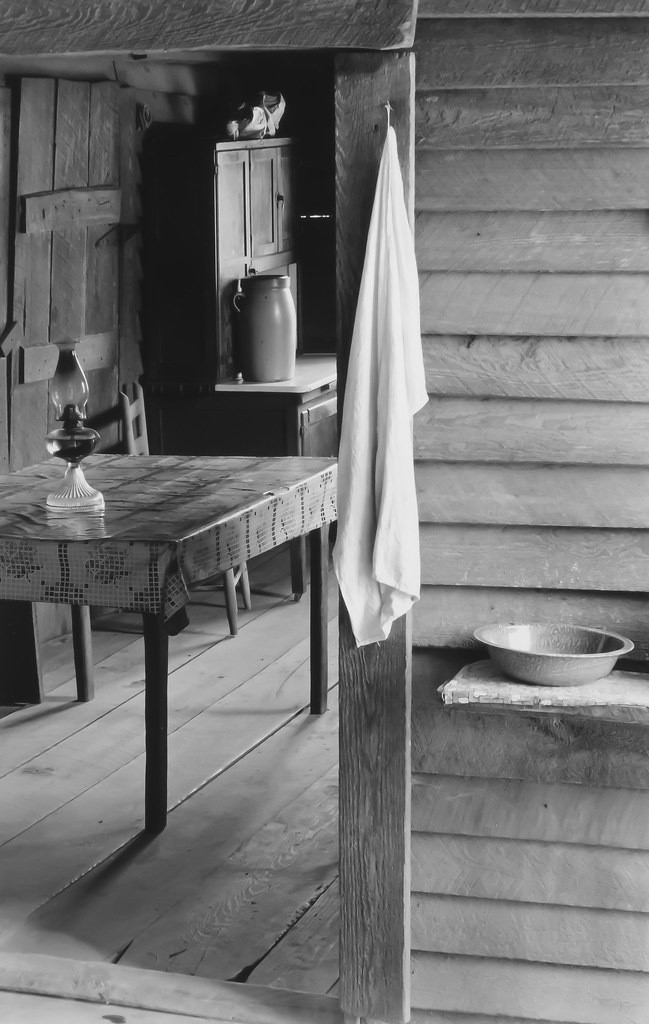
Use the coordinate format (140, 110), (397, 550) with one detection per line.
(138, 121), (338, 602)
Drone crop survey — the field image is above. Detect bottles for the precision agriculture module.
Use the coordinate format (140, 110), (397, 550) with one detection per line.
(229, 275), (297, 383)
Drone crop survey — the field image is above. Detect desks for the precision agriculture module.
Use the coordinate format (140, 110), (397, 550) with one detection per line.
(2, 454), (338, 835)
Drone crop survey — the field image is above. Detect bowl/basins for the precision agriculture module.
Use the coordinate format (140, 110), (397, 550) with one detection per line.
(473, 620), (634, 688)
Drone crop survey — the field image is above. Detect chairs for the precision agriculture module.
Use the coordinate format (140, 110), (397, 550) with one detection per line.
(117, 380), (252, 636)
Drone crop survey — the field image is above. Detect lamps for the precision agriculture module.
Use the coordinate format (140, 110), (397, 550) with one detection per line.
(43, 333), (106, 514)
